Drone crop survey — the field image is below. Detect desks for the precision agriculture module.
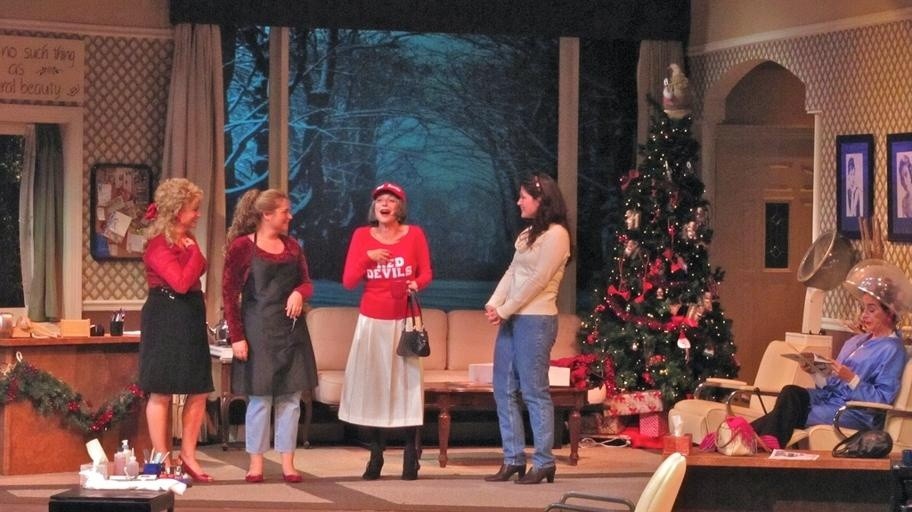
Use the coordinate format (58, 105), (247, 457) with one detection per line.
(207, 345), (321, 450)
(2, 332), (175, 472)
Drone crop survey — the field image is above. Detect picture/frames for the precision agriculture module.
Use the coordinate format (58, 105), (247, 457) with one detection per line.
(91, 162), (153, 262)
(884, 132), (912, 244)
(835, 134), (873, 238)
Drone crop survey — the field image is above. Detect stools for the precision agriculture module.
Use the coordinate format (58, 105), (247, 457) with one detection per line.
(49, 485), (174, 511)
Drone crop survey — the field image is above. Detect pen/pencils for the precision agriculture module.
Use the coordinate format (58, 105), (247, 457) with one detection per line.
(111, 308), (125, 322)
(142, 447), (170, 464)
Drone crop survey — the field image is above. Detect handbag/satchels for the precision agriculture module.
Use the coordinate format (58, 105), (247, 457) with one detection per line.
(396, 331), (430, 356)
(715, 417), (757, 457)
(832, 430), (893, 457)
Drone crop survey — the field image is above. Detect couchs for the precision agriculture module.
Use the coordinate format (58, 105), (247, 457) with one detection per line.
(302, 305), (588, 451)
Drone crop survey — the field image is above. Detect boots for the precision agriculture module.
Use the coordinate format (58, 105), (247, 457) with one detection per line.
(402, 426), (420, 479)
(362, 426), (384, 480)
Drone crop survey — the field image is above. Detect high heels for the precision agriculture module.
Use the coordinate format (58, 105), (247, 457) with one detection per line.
(174, 454), (213, 483)
(515, 467), (556, 484)
(485, 464), (526, 482)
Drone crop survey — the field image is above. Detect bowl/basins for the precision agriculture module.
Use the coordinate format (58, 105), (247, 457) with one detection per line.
(795, 230), (852, 293)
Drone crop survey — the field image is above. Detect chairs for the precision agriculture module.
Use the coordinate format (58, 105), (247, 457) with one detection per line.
(667, 340), (818, 449)
(782, 356), (910, 455)
(548, 450), (687, 511)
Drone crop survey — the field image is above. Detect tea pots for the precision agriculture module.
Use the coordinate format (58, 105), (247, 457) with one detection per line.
(206, 305), (231, 345)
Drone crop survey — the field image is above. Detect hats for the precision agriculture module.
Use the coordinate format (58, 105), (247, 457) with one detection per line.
(372, 182), (407, 202)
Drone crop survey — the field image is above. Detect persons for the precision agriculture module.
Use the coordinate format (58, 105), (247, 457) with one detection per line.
(846, 157), (863, 218)
(135, 177), (216, 485)
(222, 188), (314, 482)
(483, 173), (571, 485)
(899, 155), (911, 219)
(341, 183), (433, 480)
(749, 276), (907, 449)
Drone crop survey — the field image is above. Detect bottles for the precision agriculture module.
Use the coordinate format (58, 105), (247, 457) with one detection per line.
(113, 446), (125, 475)
(125, 446), (140, 478)
(174, 464), (181, 476)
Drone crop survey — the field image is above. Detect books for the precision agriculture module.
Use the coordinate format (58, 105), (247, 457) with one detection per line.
(781, 352), (843, 377)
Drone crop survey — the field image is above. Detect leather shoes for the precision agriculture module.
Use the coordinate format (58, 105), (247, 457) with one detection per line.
(246, 474), (264, 482)
(282, 472), (302, 483)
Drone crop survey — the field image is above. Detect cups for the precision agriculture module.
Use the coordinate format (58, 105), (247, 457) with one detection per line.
(143, 464), (162, 477)
(109, 320), (124, 336)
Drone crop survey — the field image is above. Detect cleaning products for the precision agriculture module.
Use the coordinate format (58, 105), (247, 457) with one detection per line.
(120, 438), (133, 464)
